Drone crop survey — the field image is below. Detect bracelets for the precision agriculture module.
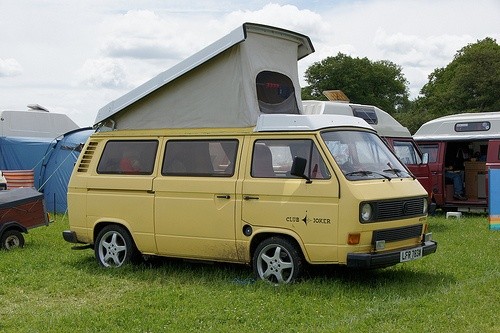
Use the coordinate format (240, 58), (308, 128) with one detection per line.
(448, 167), (449, 170)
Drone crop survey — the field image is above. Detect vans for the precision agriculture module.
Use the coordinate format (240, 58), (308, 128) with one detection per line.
(301, 102), (438, 207)
(412, 111), (500, 214)
(60, 112), (438, 288)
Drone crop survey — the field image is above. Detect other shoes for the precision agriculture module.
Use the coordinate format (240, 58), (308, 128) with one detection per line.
(453, 193), (468, 201)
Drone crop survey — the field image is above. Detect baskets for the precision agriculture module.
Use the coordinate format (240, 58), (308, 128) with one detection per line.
(471, 158), (478, 163)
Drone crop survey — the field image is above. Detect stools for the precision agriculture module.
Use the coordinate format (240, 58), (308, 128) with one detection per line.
(446, 212), (462, 220)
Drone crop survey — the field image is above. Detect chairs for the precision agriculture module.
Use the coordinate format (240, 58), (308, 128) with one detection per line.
(252, 144), (276, 177)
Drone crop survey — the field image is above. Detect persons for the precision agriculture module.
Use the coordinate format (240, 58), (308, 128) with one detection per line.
(120, 145), (144, 172)
(445, 160), (467, 201)
